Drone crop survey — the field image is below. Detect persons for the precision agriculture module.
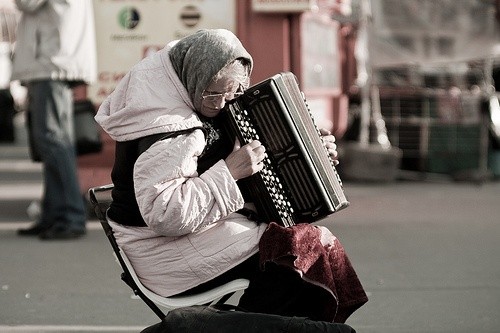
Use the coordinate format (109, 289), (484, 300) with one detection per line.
(94, 29), (368, 324)
(10, 0), (98, 241)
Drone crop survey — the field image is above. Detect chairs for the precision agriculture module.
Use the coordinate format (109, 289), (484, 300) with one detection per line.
(83, 183), (341, 323)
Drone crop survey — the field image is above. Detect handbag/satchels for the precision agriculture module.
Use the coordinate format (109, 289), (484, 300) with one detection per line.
(25, 98), (103, 161)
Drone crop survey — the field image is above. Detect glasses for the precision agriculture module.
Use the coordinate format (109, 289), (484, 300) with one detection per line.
(201, 83), (244, 102)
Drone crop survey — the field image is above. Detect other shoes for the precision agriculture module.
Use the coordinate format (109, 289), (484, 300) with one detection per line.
(40, 226), (85, 239)
(17, 224), (40, 236)
